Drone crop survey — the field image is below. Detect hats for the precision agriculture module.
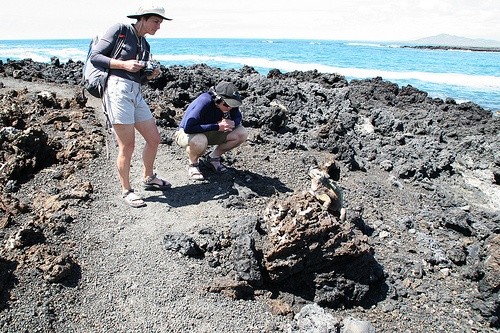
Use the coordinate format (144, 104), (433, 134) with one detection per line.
(216, 81), (242, 108)
(127, 2), (172, 20)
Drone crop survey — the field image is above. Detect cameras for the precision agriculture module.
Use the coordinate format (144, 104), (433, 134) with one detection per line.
(138, 61), (158, 74)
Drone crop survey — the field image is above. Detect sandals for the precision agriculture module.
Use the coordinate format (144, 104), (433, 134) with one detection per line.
(122, 188), (146, 208)
(186, 162), (204, 179)
(206, 154), (227, 173)
(141, 172), (172, 190)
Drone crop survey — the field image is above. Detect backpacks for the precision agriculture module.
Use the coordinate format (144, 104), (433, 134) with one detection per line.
(82, 22), (130, 98)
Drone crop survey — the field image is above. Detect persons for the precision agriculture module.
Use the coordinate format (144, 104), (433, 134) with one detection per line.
(90, 4), (173, 208)
(176, 82), (247, 181)
